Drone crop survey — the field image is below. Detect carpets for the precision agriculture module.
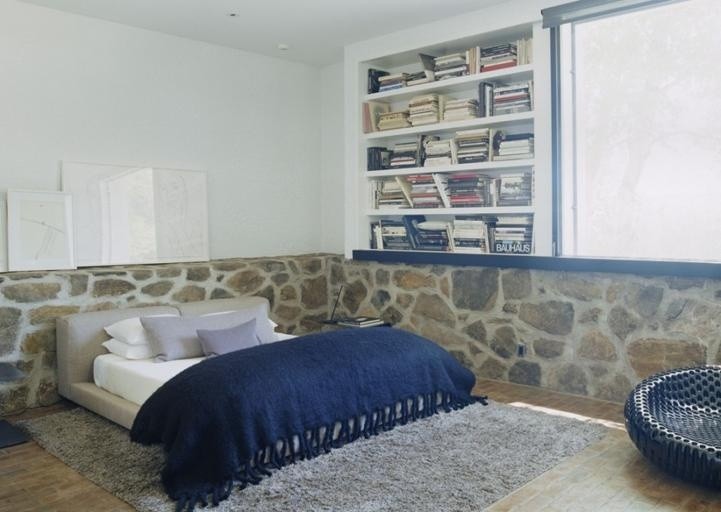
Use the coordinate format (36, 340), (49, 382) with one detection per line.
(10, 396), (611, 512)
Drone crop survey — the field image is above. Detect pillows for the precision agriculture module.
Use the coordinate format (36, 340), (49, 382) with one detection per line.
(102, 303), (278, 362)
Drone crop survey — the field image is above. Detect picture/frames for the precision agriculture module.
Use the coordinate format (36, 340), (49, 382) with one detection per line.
(7, 187), (77, 272)
(59, 160), (210, 267)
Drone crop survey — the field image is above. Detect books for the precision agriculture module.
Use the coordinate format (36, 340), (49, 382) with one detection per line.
(362, 39), (534, 254)
(338, 317), (385, 328)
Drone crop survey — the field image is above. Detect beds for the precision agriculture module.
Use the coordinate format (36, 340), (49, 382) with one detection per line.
(56, 296), (488, 512)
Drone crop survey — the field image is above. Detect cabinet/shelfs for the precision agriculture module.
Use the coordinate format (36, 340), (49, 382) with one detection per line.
(359, 21), (535, 254)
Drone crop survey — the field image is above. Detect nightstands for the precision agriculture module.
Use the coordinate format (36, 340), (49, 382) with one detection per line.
(321, 316), (391, 333)
